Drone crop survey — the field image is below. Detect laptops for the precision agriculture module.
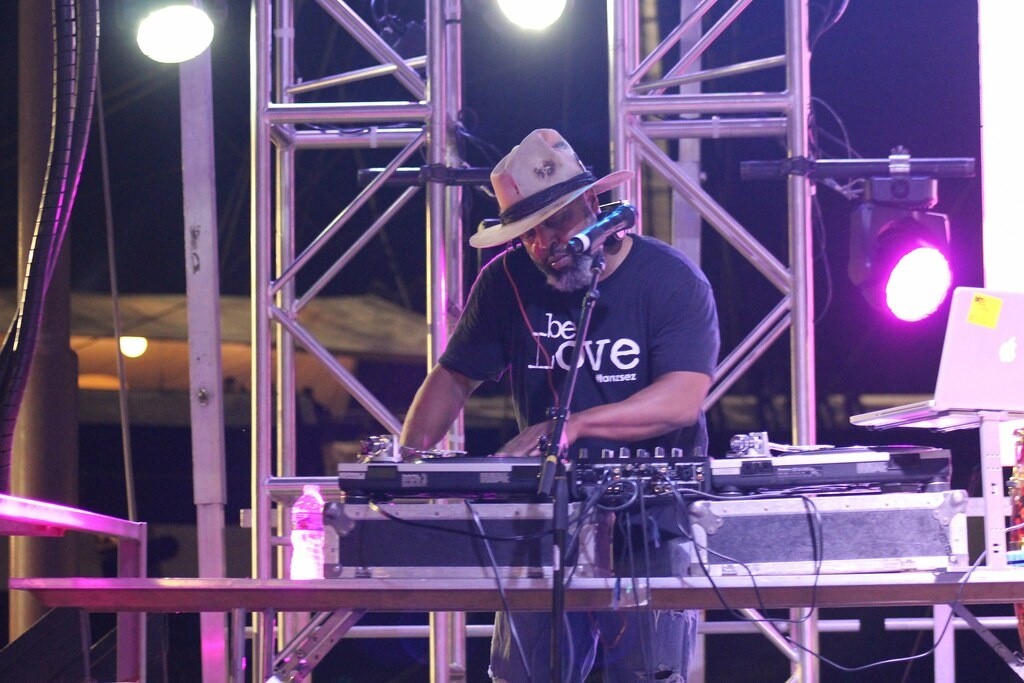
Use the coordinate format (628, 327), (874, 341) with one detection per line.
(850, 286), (1024, 428)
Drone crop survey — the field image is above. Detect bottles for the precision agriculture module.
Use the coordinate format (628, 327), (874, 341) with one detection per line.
(289, 484), (325, 579)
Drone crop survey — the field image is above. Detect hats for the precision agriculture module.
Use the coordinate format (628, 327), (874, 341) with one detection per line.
(469, 128), (636, 250)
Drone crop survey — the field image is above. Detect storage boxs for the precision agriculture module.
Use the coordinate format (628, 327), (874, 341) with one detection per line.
(662, 489), (971, 575)
(322, 501), (615, 580)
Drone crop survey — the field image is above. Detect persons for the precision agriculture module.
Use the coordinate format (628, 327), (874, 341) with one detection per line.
(400, 127), (721, 683)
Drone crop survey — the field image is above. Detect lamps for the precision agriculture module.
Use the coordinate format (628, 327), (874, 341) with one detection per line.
(848, 172), (951, 323)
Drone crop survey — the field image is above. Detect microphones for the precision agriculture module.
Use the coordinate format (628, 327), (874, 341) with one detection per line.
(565, 204), (639, 256)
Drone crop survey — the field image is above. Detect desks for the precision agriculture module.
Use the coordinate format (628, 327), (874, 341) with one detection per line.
(0, 567), (1024, 683)
